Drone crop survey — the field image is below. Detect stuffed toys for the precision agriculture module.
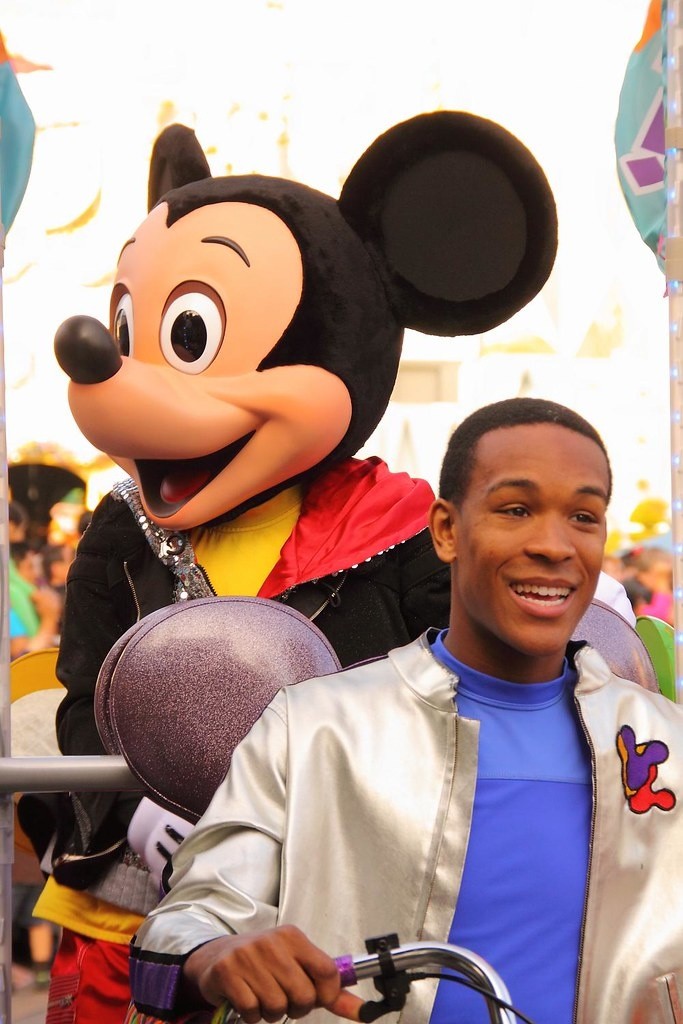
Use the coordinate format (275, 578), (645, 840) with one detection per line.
(1, 107), (562, 1022)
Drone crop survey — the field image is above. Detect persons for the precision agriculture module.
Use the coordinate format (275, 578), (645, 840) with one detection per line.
(601, 541), (673, 630)
(124, 398), (683, 1023)
(7, 486), (98, 659)
